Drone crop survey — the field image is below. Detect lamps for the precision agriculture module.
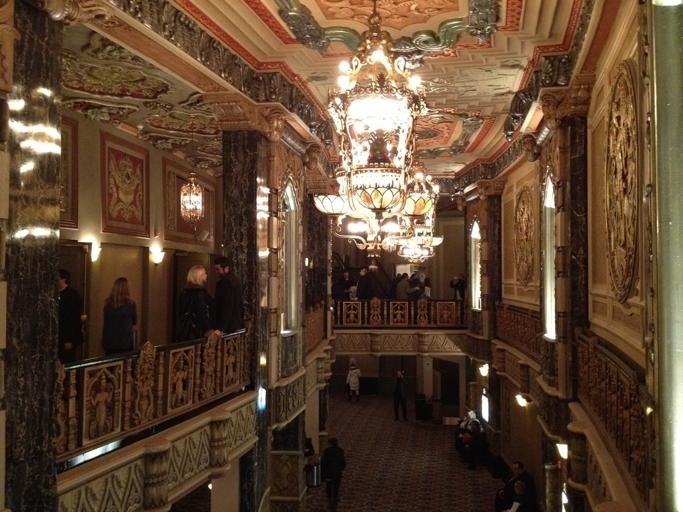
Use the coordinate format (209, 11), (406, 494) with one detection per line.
(309, 0), (445, 273)
(179, 122), (205, 227)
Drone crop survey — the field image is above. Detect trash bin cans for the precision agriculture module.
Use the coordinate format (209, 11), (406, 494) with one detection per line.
(307, 463), (321, 487)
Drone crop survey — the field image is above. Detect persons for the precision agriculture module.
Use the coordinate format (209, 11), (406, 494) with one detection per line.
(207, 255), (244, 339)
(345, 362), (361, 402)
(493, 461), (537, 511)
(454, 411), (477, 464)
(332, 265), (431, 301)
(320, 437), (346, 512)
(466, 419), (486, 472)
(391, 369), (410, 422)
(176, 263), (218, 338)
(448, 275), (466, 325)
(101, 276), (140, 355)
(57, 270), (83, 369)
(501, 480), (533, 511)
